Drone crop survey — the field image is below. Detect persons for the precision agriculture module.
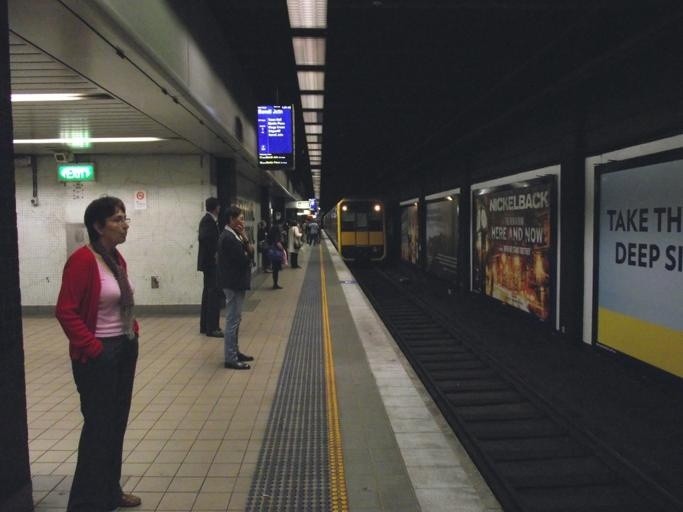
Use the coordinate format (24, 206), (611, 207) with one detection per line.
(257, 216), (322, 289)
(217, 206), (254, 370)
(54, 197), (141, 512)
(197, 197), (225, 337)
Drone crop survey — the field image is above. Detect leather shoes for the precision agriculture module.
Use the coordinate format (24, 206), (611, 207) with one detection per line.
(238, 353), (254, 361)
(224, 361), (251, 369)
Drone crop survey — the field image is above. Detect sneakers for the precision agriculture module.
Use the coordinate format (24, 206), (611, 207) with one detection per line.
(200, 328), (224, 337)
(117, 494), (143, 507)
(264, 265), (301, 289)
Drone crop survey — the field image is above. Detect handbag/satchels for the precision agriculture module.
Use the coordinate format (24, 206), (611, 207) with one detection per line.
(294, 241), (301, 249)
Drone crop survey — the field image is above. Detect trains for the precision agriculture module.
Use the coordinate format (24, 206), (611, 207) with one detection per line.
(320, 197), (385, 263)
